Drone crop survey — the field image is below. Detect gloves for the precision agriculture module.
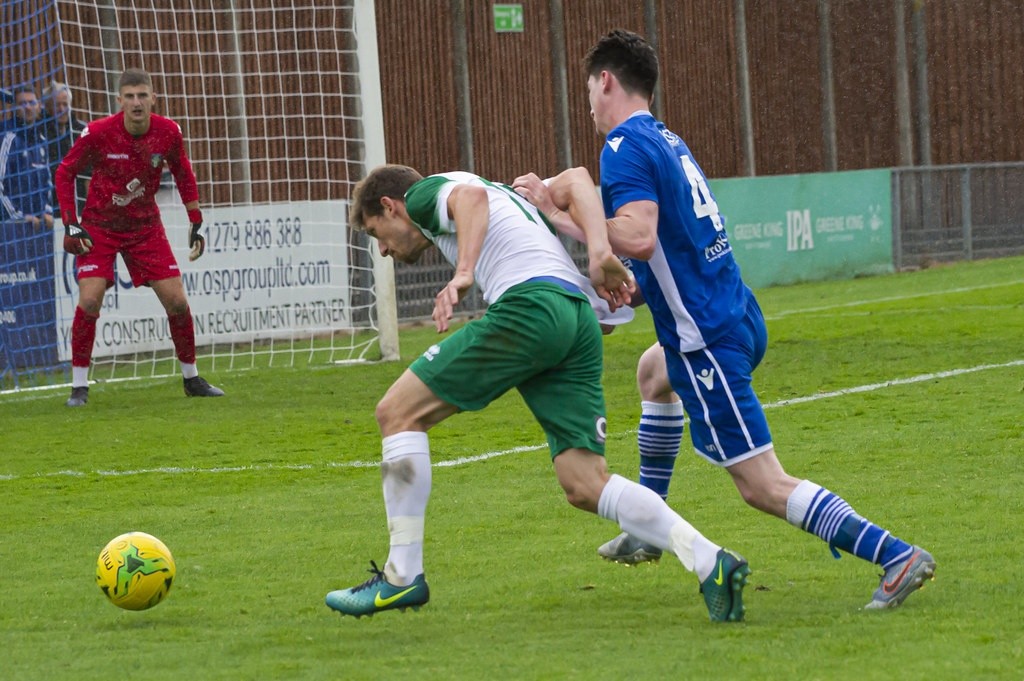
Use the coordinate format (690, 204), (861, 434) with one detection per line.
(63, 222), (94, 256)
(187, 224), (206, 260)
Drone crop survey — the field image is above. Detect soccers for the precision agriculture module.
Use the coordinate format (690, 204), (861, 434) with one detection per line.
(97, 531), (175, 611)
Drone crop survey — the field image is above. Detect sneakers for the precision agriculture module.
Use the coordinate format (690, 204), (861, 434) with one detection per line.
(65, 386), (89, 407)
(183, 375), (224, 397)
(702, 546), (754, 621)
(863, 545), (937, 609)
(598, 532), (662, 564)
(324, 570), (431, 619)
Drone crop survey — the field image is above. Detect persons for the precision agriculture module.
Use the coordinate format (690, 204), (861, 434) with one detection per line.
(40, 78), (92, 219)
(55, 67), (224, 406)
(0, 88), (13, 121)
(0, 85), (55, 314)
(325, 164), (748, 624)
(511, 27), (939, 610)
